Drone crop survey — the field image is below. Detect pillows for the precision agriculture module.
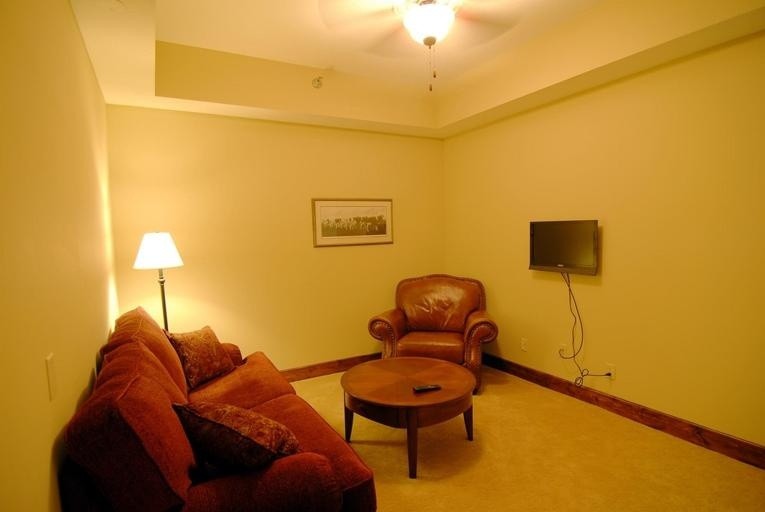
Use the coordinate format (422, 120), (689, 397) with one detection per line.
(166, 396), (303, 480)
(159, 324), (236, 388)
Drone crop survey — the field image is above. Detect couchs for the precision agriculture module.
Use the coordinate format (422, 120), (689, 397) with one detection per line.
(52, 301), (376, 512)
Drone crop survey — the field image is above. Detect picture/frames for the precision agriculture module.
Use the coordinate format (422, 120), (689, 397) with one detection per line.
(309, 193), (395, 249)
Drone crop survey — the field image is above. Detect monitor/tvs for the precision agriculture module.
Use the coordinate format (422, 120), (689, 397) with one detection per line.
(529, 220), (598, 276)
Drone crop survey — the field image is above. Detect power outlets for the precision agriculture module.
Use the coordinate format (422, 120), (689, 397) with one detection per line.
(606, 362), (617, 381)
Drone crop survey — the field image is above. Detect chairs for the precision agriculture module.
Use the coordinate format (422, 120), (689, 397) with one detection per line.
(367, 273), (499, 395)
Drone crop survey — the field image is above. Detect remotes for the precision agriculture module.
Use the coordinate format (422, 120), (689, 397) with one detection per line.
(413, 385), (441, 392)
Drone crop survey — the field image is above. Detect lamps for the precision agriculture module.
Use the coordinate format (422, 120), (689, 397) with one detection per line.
(132, 231), (188, 332)
(395, 1), (461, 92)
